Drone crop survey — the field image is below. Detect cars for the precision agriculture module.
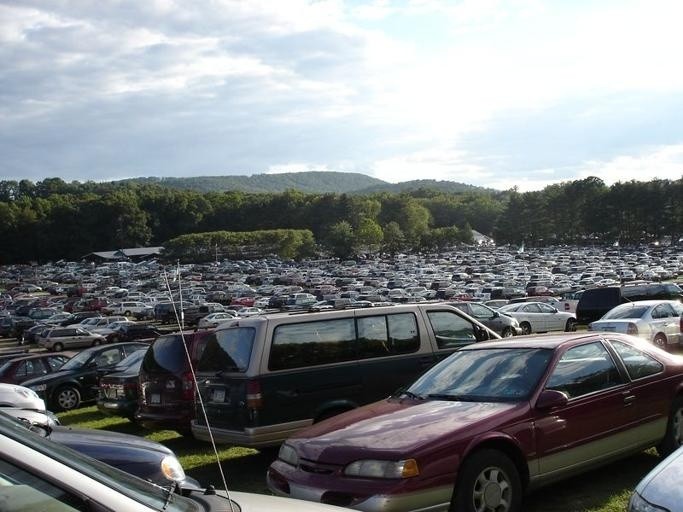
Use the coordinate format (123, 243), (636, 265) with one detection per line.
(0, 328), (150, 422)
